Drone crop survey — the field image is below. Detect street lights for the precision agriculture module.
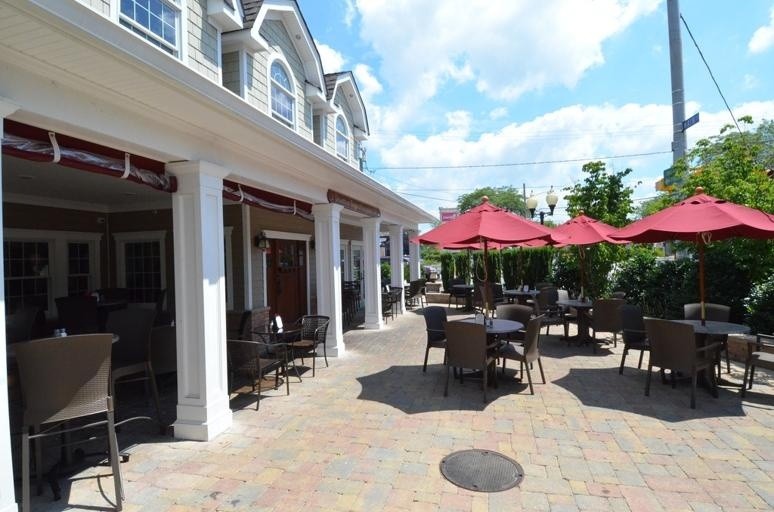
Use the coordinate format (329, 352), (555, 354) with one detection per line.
(528, 184), (558, 226)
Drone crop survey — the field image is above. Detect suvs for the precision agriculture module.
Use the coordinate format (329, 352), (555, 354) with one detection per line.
(380, 255), (440, 283)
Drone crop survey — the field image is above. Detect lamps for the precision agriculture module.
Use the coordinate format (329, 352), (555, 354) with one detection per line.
(256, 232), (269, 251)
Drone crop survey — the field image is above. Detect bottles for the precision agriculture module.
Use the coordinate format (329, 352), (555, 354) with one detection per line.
(53, 328), (66, 337)
(488, 317), (493, 328)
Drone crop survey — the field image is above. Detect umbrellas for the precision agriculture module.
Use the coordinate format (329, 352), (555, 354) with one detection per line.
(605, 185), (774, 327)
(442, 219), (560, 251)
(547, 209), (635, 302)
(407, 195), (553, 316)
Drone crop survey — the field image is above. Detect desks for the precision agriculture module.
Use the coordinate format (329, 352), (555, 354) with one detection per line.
(249, 321), (305, 382)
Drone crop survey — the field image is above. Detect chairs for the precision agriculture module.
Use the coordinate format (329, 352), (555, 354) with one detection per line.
(227, 339), (290, 412)
(90, 287), (129, 320)
(227, 310), (250, 344)
(9, 303), (39, 344)
(52, 293), (98, 331)
(341, 272), (426, 330)
(421, 271), (772, 409)
(7, 333), (128, 512)
(7, 272), (179, 434)
(289, 315), (331, 377)
(94, 308), (161, 415)
